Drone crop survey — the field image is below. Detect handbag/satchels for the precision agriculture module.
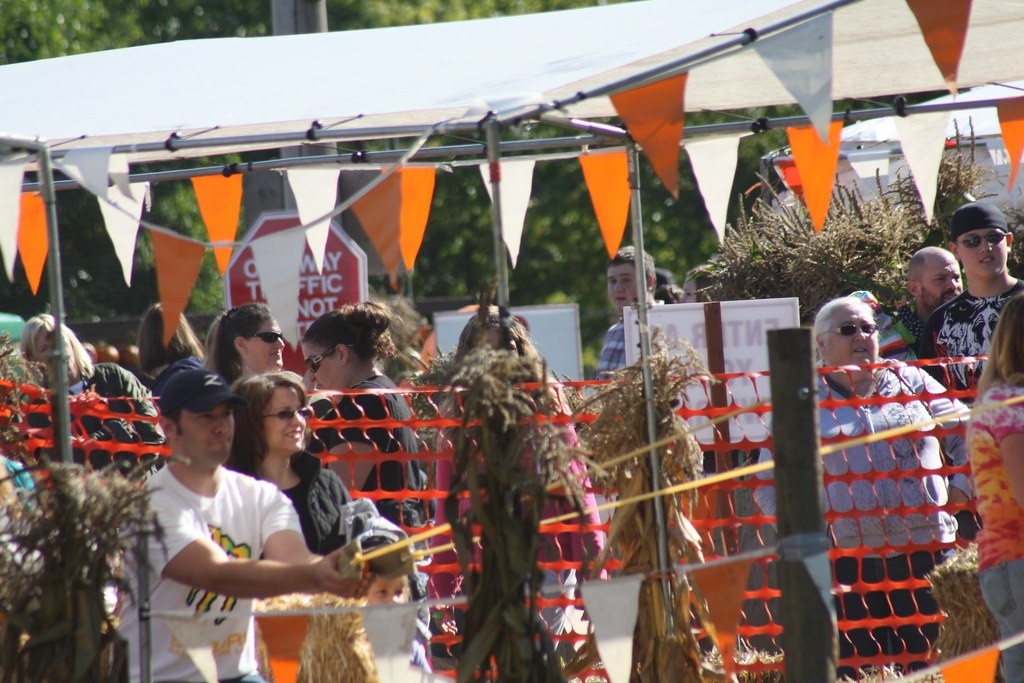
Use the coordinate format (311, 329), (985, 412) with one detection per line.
(944, 453), (983, 540)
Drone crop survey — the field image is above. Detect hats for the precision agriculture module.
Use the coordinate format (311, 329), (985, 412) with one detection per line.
(655, 269), (673, 288)
(160, 369), (245, 416)
(950, 203), (1008, 242)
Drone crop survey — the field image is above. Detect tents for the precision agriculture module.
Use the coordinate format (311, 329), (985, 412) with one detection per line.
(760, 81), (1024, 212)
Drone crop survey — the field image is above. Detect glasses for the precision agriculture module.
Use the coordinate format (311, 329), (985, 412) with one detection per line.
(305, 344), (352, 374)
(958, 231), (1008, 248)
(833, 324), (879, 336)
(243, 331), (283, 343)
(261, 407), (312, 420)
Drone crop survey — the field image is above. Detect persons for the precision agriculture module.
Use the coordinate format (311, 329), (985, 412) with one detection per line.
(140, 303), (610, 683)
(21, 314), (166, 485)
(754, 202), (1024, 683)
(595, 246), (714, 380)
(0, 454), (45, 577)
